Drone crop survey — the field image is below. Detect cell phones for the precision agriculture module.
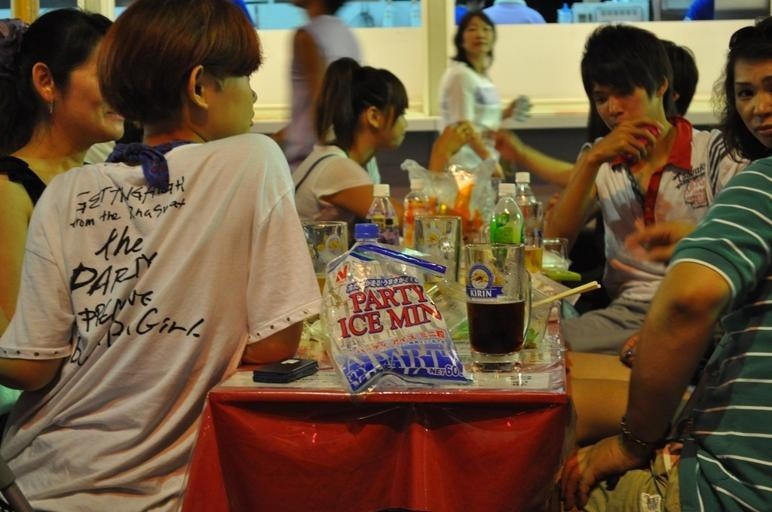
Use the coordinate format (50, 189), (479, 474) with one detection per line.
(253, 357), (318, 383)
(611, 124), (658, 171)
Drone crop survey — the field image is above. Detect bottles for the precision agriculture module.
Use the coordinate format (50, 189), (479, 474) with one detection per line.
(403, 180), (429, 245)
(510, 96), (530, 122)
(487, 183), (523, 271)
(510, 173), (542, 274)
(365, 184), (401, 248)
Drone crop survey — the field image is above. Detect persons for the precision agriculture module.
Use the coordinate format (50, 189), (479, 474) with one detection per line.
(257, 1), (366, 174)
(557, 154), (771, 512)
(0, 1), (329, 512)
(537, 23), (752, 353)
(436, 12), (534, 193)
(0, 9), (129, 338)
(556, 13), (771, 450)
(490, 39), (698, 192)
(285, 59), (473, 236)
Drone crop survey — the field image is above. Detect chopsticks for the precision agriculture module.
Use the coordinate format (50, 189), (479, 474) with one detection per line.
(532, 279), (602, 309)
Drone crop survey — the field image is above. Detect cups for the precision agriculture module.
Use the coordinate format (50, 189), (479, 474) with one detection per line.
(299, 219), (348, 292)
(462, 241), (528, 372)
(541, 238), (568, 270)
(412, 213), (462, 288)
(351, 225), (383, 254)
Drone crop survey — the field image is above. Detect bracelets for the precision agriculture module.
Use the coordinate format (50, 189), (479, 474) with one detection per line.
(618, 415), (672, 451)
